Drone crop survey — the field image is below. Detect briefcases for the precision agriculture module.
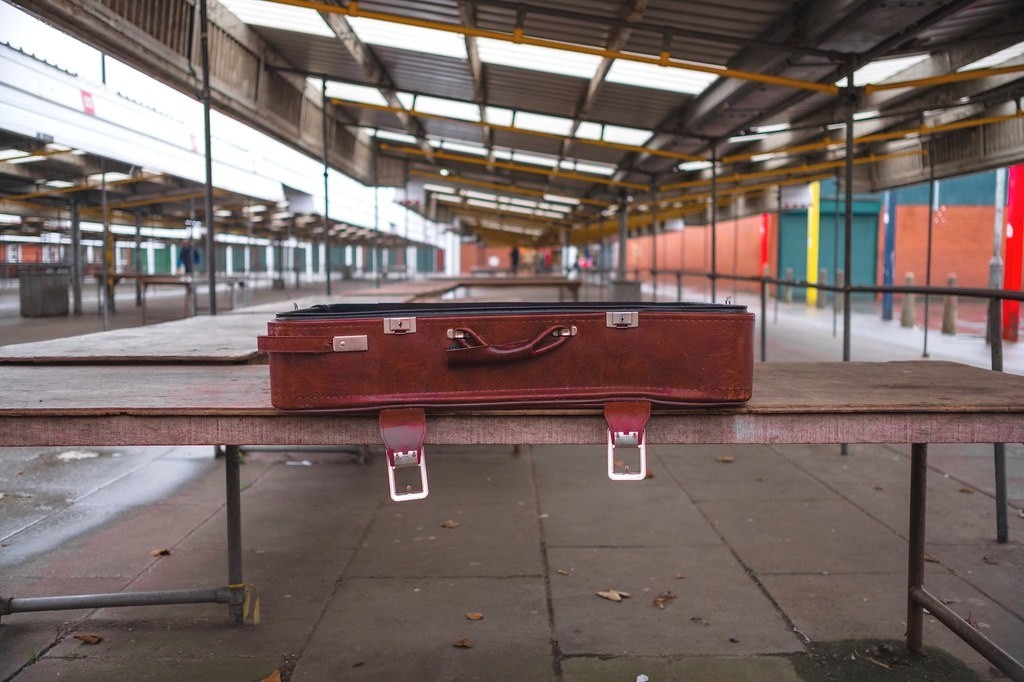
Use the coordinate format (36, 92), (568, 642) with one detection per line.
(255, 303), (755, 500)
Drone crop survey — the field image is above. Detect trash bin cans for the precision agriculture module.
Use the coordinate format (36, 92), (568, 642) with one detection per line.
(606, 278), (642, 304)
(16, 262), (71, 317)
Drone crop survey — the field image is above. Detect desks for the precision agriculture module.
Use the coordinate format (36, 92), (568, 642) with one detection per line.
(0, 310), (377, 468)
(0, 360), (1023, 681)
(92, 265), (642, 331)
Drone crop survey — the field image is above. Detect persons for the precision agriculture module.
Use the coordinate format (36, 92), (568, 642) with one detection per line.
(176, 240), (199, 294)
(509, 247), (520, 274)
(533, 250), (599, 274)
